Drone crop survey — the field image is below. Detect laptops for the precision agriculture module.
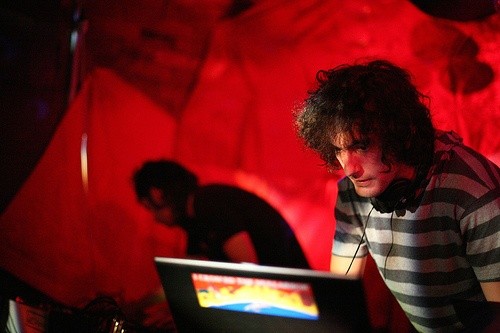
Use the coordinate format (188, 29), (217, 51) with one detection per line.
(154, 257), (373, 333)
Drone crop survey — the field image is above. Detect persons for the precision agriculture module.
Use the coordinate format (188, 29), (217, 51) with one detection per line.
(129, 155), (313, 271)
(294, 57), (500, 333)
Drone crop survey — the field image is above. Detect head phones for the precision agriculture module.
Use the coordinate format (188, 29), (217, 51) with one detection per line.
(370, 109), (435, 213)
